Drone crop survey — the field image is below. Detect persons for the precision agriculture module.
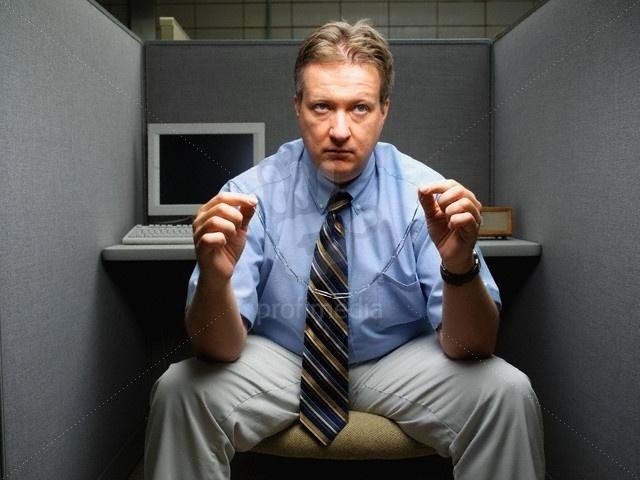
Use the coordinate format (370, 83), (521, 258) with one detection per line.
(142, 17), (547, 480)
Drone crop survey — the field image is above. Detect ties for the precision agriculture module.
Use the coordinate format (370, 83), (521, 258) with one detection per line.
(300, 192), (351, 452)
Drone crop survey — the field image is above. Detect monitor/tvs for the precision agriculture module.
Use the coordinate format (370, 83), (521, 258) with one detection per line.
(147, 123), (265, 216)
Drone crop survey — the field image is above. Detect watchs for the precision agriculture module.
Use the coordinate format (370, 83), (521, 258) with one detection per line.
(439, 250), (481, 286)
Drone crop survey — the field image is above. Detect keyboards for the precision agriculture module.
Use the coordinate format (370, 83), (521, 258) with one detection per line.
(121, 224), (194, 245)
